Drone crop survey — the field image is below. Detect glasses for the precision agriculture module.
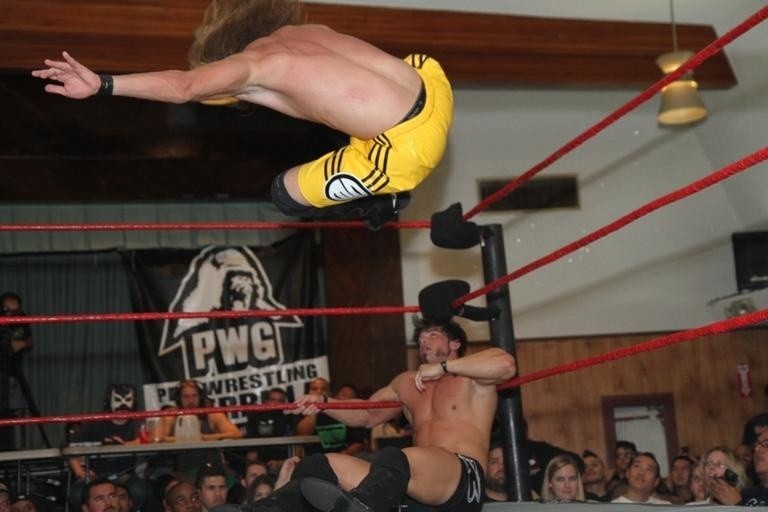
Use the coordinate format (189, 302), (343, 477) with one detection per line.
(752, 439), (768, 452)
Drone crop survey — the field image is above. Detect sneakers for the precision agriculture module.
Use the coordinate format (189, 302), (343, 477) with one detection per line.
(209, 504), (281, 512)
(299, 477), (369, 512)
(393, 192), (412, 210)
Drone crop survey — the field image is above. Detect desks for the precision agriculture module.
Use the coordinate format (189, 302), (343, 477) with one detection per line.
(63, 435), (321, 479)
(0, 447), (61, 512)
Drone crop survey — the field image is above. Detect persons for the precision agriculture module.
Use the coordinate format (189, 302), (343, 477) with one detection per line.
(1, 293), (767, 510)
(32, 0), (455, 231)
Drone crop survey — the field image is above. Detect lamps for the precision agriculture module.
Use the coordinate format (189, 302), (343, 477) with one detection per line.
(657, 1), (708, 125)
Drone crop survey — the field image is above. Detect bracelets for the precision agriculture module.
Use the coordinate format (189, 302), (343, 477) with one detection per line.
(96, 73), (113, 96)
